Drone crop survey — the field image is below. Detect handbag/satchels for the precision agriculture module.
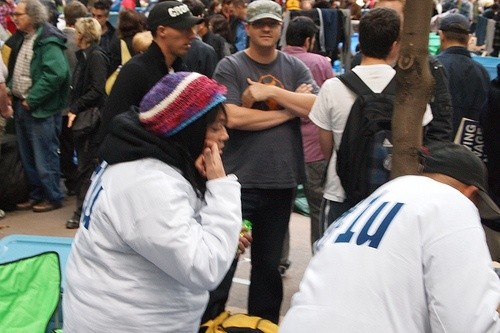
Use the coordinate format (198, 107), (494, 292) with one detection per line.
(105, 40), (132, 96)
(71, 107), (101, 141)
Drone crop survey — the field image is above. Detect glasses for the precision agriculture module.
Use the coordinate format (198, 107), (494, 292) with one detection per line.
(8, 12), (27, 17)
(248, 22), (281, 27)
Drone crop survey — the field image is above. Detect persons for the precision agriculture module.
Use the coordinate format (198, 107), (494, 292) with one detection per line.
(59, 72), (252, 333)
(307, 8), (433, 228)
(277, 16), (336, 273)
(274, 0), (405, 64)
(101, 0), (204, 144)
(170, 0), (250, 78)
(278, 142), (500, 333)
(421, 0), (500, 209)
(0, 0), (155, 229)
(198, 0), (321, 333)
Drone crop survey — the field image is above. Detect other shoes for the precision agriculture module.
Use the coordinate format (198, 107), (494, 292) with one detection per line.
(0, 210), (4, 217)
(281, 267), (288, 275)
(17, 200), (39, 210)
(33, 200), (61, 211)
(67, 212), (79, 228)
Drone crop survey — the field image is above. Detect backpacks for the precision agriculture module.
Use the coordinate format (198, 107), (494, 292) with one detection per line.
(336, 69), (405, 204)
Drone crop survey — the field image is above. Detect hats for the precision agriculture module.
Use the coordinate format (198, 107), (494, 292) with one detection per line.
(246, 1), (282, 22)
(436, 14), (476, 36)
(286, 16), (320, 40)
(148, 1), (204, 29)
(138, 72), (228, 138)
(418, 143), (500, 219)
